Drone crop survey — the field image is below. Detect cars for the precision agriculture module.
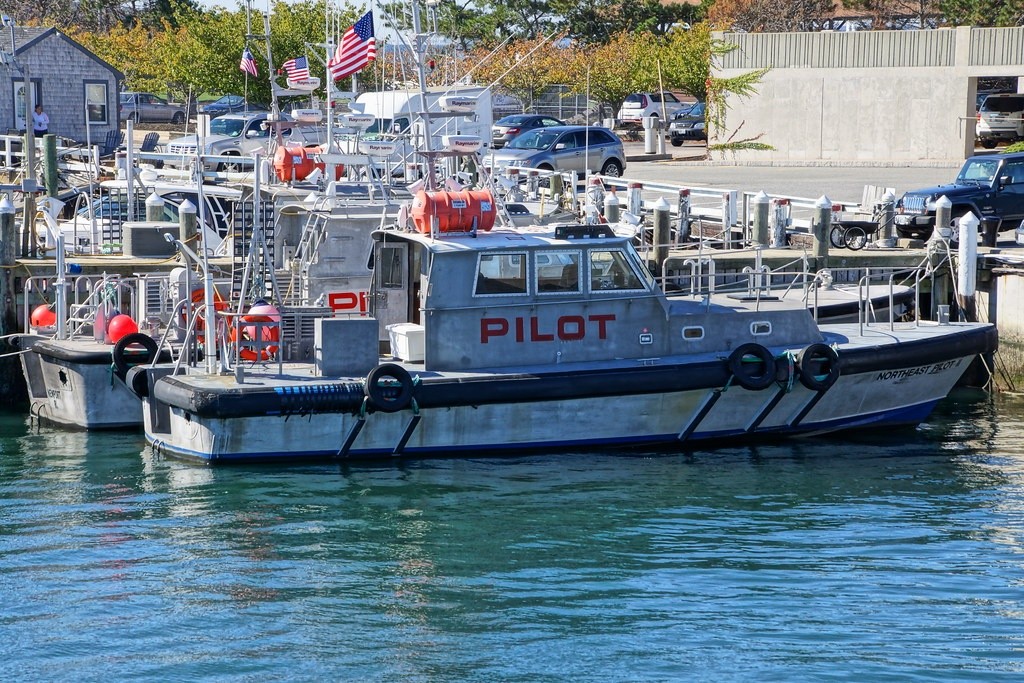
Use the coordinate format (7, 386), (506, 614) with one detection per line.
(617, 91), (681, 126)
(120, 92), (190, 124)
(894, 152), (1024, 248)
(199, 93), (268, 115)
(975, 93), (1024, 149)
(492, 114), (566, 148)
(669, 100), (699, 124)
(668, 102), (708, 146)
(482, 126), (626, 188)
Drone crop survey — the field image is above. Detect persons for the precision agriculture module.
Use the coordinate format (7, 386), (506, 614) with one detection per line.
(31, 103), (47, 137)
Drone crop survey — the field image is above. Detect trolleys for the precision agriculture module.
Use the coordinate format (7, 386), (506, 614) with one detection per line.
(830, 211), (898, 251)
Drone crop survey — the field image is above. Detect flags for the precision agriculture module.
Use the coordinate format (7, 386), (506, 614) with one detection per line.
(284, 55), (308, 81)
(240, 48), (258, 77)
(328, 9), (376, 81)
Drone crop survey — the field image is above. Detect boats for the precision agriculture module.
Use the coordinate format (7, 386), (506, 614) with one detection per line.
(126, 188), (999, 467)
(0, 180), (915, 433)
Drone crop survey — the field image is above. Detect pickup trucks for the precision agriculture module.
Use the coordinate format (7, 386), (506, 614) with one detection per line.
(167, 113), (337, 172)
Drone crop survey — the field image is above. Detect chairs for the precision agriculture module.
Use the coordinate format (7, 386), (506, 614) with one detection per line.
(139, 132), (159, 152)
(100, 130), (125, 159)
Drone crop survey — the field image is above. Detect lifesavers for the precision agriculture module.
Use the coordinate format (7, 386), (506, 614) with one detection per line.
(727, 342), (775, 390)
(798, 342), (840, 390)
(232, 317), (279, 360)
(363, 363), (414, 411)
(182, 289), (231, 345)
(113, 332), (157, 379)
